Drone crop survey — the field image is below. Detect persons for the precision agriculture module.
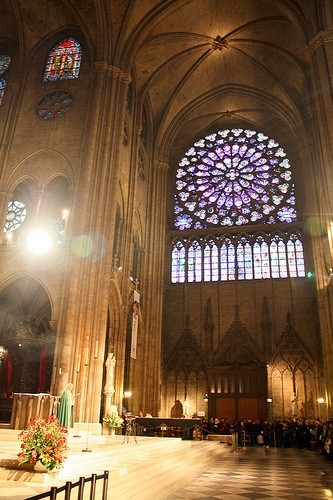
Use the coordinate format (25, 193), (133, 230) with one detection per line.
(199, 417), (333, 459)
(61, 383), (76, 429)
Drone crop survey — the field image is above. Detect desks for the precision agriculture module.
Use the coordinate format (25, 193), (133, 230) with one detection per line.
(135, 417), (201, 440)
(207, 435), (232, 447)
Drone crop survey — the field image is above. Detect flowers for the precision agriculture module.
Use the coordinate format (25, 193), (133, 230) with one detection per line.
(103, 410), (125, 429)
(15, 414), (69, 474)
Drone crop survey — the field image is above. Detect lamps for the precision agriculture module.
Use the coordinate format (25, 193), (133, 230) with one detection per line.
(203, 396), (209, 403)
(267, 397), (273, 403)
